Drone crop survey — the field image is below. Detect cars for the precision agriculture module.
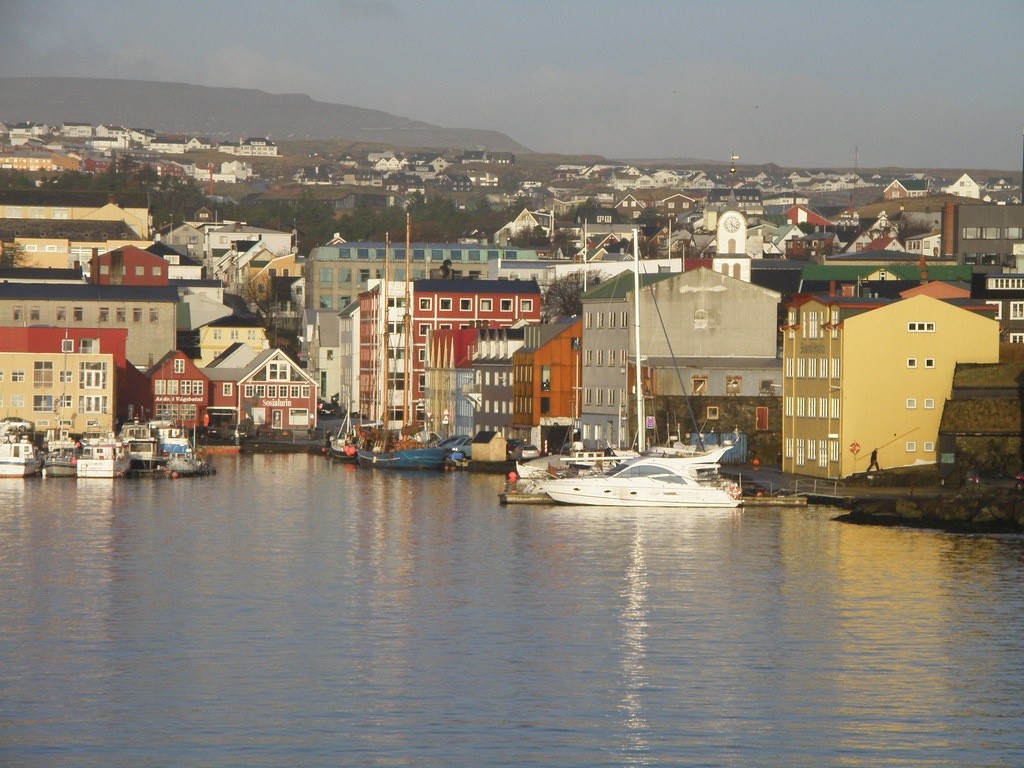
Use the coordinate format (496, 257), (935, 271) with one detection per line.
(320, 403), (336, 417)
(221, 423), (247, 441)
(207, 422), (221, 439)
(520, 444), (541, 460)
(432, 434), (473, 460)
(506, 437), (524, 458)
(330, 393), (339, 402)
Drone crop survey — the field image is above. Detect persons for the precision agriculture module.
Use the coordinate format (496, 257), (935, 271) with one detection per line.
(776, 452), (782, 469)
(866, 448), (880, 472)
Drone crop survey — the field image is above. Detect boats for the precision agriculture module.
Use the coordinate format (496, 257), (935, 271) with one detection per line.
(76, 413), (208, 478)
(0, 423), (39, 480)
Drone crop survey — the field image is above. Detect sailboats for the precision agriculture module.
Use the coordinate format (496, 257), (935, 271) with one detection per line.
(326, 212), (449, 472)
(43, 319), (78, 477)
(517, 227), (747, 508)
